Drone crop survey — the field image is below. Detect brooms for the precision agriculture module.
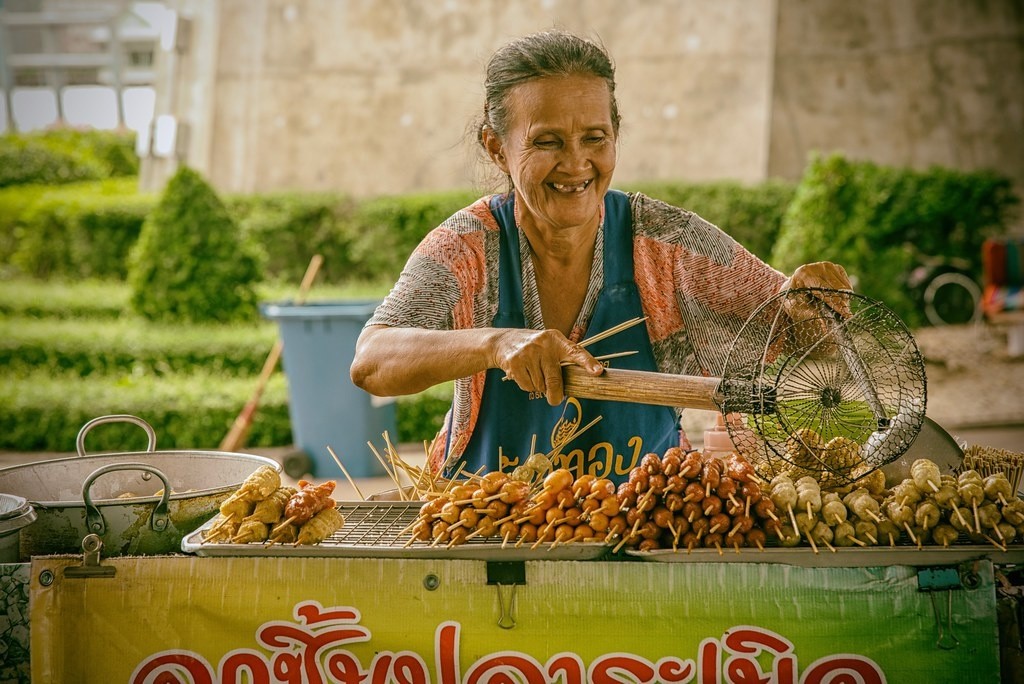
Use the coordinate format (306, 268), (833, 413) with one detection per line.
(212, 250), (324, 452)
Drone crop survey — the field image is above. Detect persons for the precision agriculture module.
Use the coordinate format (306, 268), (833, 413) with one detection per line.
(349, 31), (852, 479)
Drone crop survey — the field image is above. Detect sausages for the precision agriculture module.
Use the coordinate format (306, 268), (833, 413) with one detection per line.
(414, 467), (628, 542)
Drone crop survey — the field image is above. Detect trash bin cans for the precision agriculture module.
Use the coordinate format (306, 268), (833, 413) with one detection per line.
(263, 302), (397, 480)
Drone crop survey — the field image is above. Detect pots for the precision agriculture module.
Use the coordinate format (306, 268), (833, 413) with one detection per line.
(0, 414), (283, 562)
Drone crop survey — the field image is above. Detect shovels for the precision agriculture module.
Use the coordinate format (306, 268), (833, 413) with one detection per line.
(817, 295), (964, 490)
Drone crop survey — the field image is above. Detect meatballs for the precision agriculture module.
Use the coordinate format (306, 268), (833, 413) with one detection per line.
(614, 429), (1024, 546)
